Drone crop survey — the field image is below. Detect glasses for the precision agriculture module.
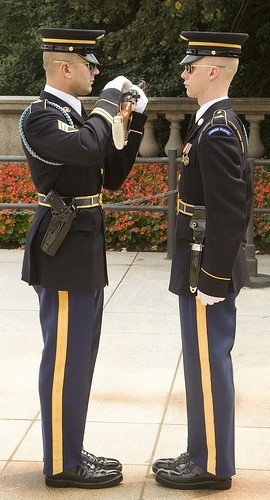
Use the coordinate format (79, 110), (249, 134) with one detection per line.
(184, 63), (217, 75)
(53, 60), (95, 70)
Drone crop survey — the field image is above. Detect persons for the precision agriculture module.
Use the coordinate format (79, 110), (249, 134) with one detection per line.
(151, 31), (253, 491)
(18, 28), (149, 489)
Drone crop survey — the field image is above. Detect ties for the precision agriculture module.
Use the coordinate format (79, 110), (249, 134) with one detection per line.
(186, 113), (196, 135)
(81, 102), (89, 121)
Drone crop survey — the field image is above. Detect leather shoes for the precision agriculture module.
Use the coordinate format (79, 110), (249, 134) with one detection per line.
(46, 449), (124, 489)
(152, 452), (232, 490)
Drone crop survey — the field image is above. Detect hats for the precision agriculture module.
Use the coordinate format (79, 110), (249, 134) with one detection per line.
(179, 31), (249, 65)
(38, 28), (105, 65)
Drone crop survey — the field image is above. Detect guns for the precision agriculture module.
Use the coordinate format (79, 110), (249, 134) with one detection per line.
(40, 190), (78, 257)
(112, 77), (150, 150)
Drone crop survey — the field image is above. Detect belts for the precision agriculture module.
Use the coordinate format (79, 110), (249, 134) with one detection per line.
(38, 193), (102, 208)
(177, 199), (194, 216)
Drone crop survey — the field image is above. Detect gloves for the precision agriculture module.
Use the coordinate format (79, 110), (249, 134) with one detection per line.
(102, 75), (133, 94)
(197, 289), (226, 305)
(129, 84), (148, 115)
